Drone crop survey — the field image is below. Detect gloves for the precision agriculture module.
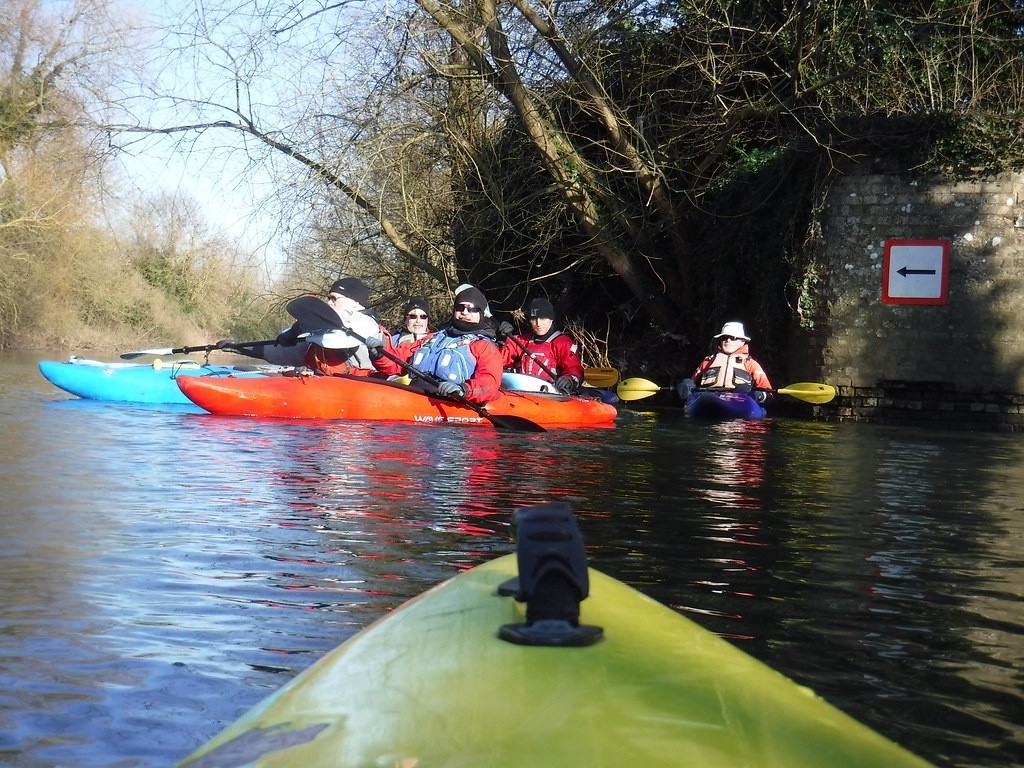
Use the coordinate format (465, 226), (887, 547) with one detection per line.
(555, 377), (571, 390)
(438, 380), (464, 401)
(495, 321), (514, 342)
(217, 340), (246, 354)
(274, 330), (299, 347)
(366, 337), (382, 359)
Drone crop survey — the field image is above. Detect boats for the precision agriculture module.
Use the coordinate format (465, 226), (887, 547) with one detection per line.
(169, 504), (932, 768)
(683, 387), (770, 420)
(175, 369), (618, 430)
(38, 356), (291, 405)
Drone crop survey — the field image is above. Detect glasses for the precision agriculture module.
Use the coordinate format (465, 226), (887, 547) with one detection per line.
(456, 304), (480, 312)
(327, 295), (347, 302)
(721, 335), (741, 341)
(407, 314), (427, 319)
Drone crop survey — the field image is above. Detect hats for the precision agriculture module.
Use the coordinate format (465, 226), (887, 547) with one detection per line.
(714, 322), (751, 342)
(454, 287), (487, 313)
(329, 278), (368, 308)
(526, 298), (554, 322)
(405, 298), (430, 322)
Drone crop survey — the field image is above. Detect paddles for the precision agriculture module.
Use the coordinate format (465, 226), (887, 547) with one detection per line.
(454, 282), (572, 395)
(583, 366), (619, 388)
(119, 312), (380, 361)
(285, 295), (549, 433)
(617, 376), (836, 405)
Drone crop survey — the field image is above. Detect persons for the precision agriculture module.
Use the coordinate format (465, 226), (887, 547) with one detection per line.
(676, 322), (773, 404)
(496, 298), (584, 392)
(391, 298), (430, 348)
(366, 287), (503, 403)
(217, 278), (401, 380)
